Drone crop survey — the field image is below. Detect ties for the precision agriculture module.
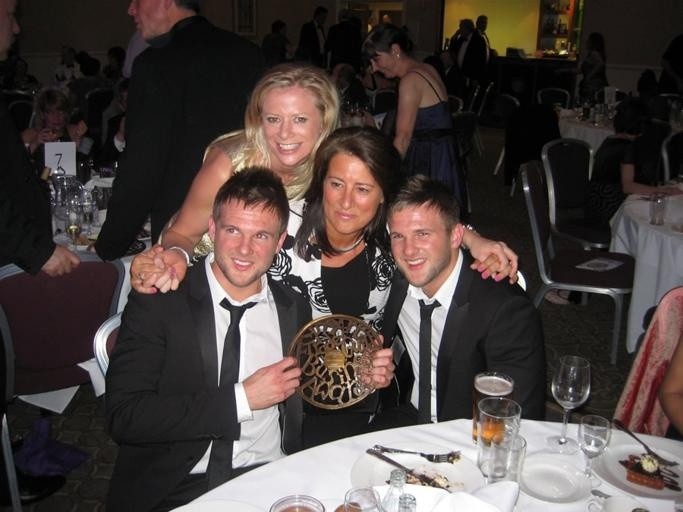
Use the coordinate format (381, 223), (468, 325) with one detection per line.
(416, 298), (440, 426)
(206, 297), (257, 486)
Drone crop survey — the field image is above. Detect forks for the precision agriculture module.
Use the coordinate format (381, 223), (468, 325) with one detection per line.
(373, 443), (459, 463)
(613, 417), (680, 467)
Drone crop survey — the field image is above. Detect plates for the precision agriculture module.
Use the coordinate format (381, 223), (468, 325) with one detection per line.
(350, 440), (483, 506)
(518, 450), (592, 502)
(601, 443), (683, 497)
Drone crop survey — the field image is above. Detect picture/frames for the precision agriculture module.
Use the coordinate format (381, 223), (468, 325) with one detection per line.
(233, 0), (257, 37)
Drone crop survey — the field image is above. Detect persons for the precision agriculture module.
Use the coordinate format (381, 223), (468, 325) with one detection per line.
(142, 63), (518, 293)
(87, 0), (276, 262)
(323, 10), (368, 75)
(129, 125), (534, 455)
(579, 32), (609, 104)
(363, 22), (469, 211)
(469, 16), (498, 76)
(361, 175), (545, 436)
(638, 69), (672, 123)
(450, 19), (475, 73)
(70, 57), (112, 97)
(261, 19), (292, 67)
(16, 56), (43, 91)
(107, 165), (396, 511)
(105, 46), (127, 87)
(659, 331), (682, 442)
(658, 35), (682, 94)
(586, 100), (683, 239)
(26, 90), (100, 178)
(293, 6), (328, 68)
(94, 78), (129, 173)
(0, 0), (81, 505)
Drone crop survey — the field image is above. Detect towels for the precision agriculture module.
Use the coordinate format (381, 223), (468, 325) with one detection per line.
(372, 478), (502, 512)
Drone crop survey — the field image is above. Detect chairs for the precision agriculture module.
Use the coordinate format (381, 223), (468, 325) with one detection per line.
(457, 83), (482, 159)
(661, 130), (683, 182)
(92, 310), (123, 375)
(85, 88), (113, 112)
(477, 83), (494, 153)
(536, 88), (571, 112)
(509, 176), (517, 198)
(518, 161), (636, 365)
(373, 90), (396, 111)
(659, 93), (680, 102)
(595, 86), (627, 105)
(0, 252), (126, 512)
(0, 89), (32, 106)
(8, 101), (32, 132)
(613, 286), (683, 439)
(541, 137), (613, 306)
(492, 94), (521, 176)
(445, 95), (461, 115)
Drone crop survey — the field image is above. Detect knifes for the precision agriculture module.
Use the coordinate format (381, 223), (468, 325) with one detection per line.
(365, 447), (451, 493)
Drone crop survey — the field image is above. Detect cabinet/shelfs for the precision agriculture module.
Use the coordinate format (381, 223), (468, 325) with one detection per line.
(536, 0), (581, 58)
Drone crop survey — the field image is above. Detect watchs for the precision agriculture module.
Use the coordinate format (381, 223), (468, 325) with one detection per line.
(456, 221), (477, 249)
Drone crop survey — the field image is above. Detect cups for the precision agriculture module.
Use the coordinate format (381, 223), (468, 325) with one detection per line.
(472, 370), (516, 445)
(648, 192), (667, 225)
(476, 397), (522, 478)
(347, 100), (371, 117)
(546, 354), (592, 456)
(269, 494), (325, 512)
(344, 484), (380, 512)
(574, 414), (612, 484)
(66, 209), (84, 253)
(486, 433), (527, 484)
(594, 113), (606, 128)
(81, 203), (94, 237)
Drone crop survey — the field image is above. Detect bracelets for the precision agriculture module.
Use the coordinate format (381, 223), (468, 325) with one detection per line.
(165, 246), (193, 265)
